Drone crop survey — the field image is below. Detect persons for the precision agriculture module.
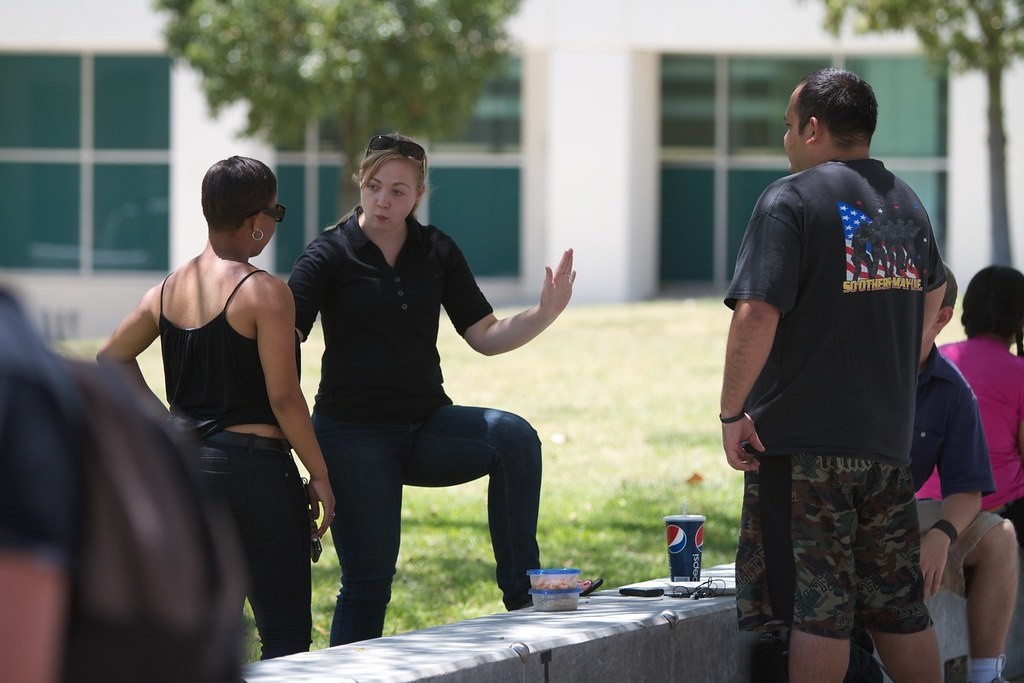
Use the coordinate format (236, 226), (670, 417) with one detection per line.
(842, 261), (1021, 682)
(909, 264), (1023, 573)
(719, 65), (949, 683)
(2, 280), (104, 683)
(93, 150), (335, 661)
(286, 131), (605, 647)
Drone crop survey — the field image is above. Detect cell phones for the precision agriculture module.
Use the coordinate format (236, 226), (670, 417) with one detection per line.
(619, 587), (664, 596)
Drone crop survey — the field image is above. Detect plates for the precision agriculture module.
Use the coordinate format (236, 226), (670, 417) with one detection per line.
(665, 578), (736, 595)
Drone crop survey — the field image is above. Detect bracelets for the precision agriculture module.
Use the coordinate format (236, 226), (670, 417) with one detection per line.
(927, 519), (959, 544)
(720, 410), (746, 424)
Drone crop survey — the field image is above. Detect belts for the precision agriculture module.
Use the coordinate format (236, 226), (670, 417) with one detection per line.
(205, 429), (293, 455)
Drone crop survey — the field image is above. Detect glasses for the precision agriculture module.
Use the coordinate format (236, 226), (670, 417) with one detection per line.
(239, 204), (286, 223)
(364, 134), (427, 177)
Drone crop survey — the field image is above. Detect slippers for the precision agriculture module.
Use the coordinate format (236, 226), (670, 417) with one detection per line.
(577, 575), (604, 597)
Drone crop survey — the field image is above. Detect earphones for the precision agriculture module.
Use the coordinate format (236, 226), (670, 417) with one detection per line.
(690, 589), (703, 600)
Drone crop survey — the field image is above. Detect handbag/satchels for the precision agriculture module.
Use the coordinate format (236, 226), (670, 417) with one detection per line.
(752, 624), (884, 683)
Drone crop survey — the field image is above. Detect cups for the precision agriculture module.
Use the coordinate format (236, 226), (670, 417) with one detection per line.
(663, 511), (707, 581)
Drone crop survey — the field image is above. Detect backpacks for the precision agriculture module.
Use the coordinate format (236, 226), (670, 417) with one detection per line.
(47, 349), (255, 683)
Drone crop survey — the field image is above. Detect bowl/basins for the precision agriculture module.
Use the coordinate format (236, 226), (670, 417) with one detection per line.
(527, 566), (580, 590)
(528, 587), (582, 611)
